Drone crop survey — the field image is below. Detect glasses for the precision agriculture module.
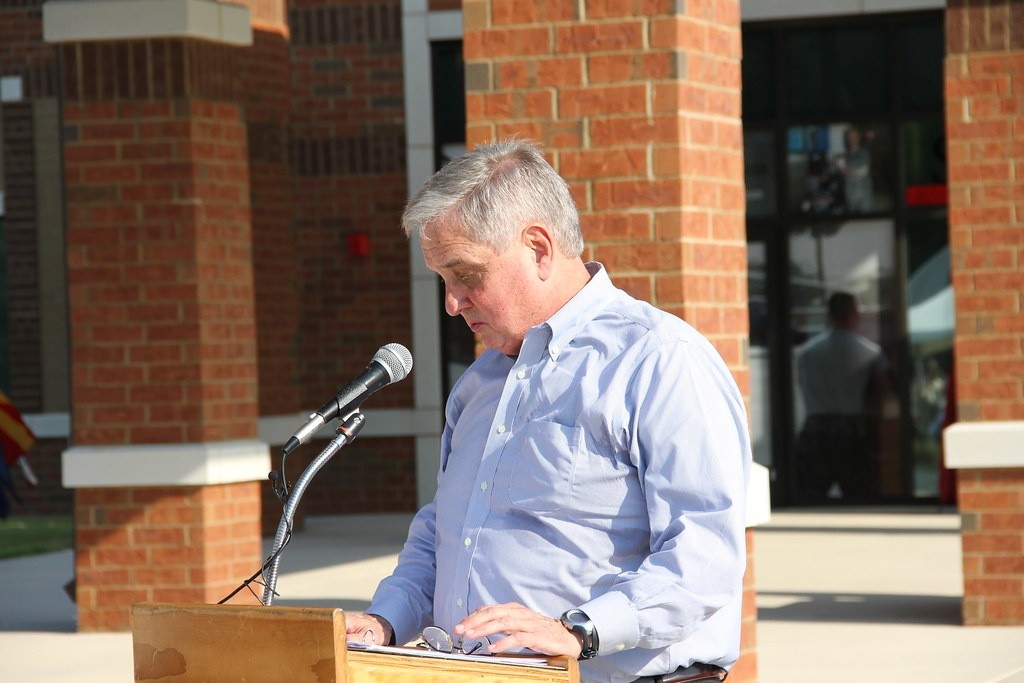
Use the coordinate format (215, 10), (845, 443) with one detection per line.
(416, 626), (496, 656)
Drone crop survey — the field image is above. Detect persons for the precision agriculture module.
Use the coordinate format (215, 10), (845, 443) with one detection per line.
(346, 134), (753, 683)
(835, 128), (872, 201)
(791, 292), (883, 429)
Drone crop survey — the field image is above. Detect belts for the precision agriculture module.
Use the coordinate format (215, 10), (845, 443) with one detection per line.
(633, 662), (727, 683)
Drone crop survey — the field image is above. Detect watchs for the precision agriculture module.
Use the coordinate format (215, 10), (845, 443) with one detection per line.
(560, 608), (598, 661)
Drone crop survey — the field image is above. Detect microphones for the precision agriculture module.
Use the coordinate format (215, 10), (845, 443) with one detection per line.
(283, 342), (412, 454)
